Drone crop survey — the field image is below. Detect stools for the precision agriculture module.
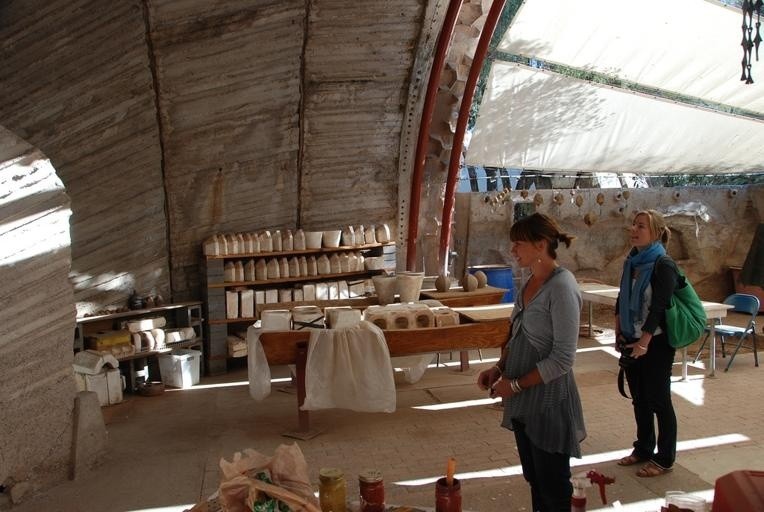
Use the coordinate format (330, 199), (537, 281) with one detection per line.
(432, 346), (486, 372)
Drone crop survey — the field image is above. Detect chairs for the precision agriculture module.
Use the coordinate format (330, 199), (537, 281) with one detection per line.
(689, 291), (761, 374)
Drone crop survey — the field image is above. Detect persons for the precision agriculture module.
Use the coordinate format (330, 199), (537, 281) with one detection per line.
(473, 212), (587, 511)
(615, 209), (678, 478)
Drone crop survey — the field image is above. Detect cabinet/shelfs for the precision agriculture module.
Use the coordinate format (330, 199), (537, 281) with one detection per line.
(72, 299), (205, 364)
(204, 239), (396, 361)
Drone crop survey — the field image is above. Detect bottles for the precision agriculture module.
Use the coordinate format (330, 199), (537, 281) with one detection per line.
(201, 222), (390, 283)
(435, 270), (487, 293)
(128, 291), (164, 309)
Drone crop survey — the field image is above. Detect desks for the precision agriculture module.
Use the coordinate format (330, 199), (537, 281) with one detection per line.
(254, 306), (514, 447)
(728, 265), (763, 316)
(419, 285), (511, 305)
(573, 276), (735, 383)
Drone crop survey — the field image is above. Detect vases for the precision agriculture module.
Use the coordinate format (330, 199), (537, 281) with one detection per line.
(370, 275), (396, 305)
(322, 230), (341, 247)
(304, 231), (323, 249)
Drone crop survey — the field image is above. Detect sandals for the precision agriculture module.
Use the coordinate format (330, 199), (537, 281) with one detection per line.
(617, 455), (646, 466)
(635, 460), (674, 478)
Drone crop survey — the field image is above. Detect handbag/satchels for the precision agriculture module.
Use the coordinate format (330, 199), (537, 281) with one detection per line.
(652, 254), (708, 351)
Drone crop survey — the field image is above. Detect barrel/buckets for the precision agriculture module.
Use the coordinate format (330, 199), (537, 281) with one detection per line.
(466, 263), (514, 303)
(202, 224), (391, 283)
(371, 273), (398, 303)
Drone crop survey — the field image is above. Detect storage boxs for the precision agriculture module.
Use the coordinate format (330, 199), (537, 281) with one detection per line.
(156, 350), (203, 390)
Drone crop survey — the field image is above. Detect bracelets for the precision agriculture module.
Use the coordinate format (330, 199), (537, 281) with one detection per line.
(491, 364), (504, 377)
(637, 340), (649, 351)
(511, 376), (522, 393)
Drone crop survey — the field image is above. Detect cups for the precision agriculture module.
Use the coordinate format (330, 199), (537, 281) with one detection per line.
(662, 489), (707, 512)
(371, 273), (424, 305)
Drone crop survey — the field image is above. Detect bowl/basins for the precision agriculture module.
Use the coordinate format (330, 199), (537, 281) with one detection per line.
(137, 380), (166, 395)
